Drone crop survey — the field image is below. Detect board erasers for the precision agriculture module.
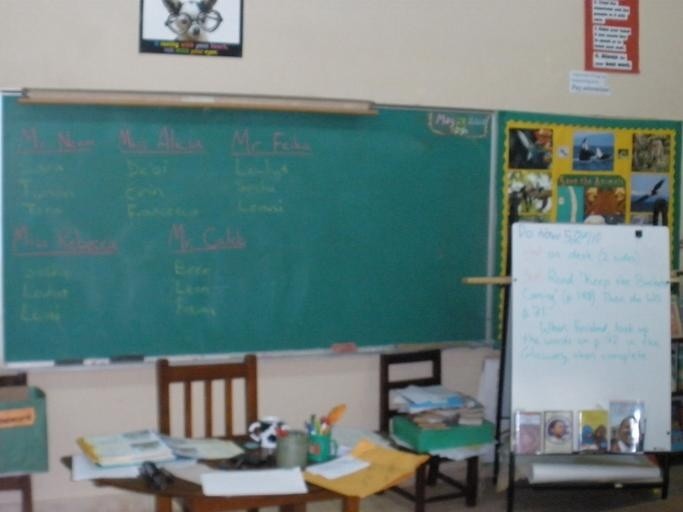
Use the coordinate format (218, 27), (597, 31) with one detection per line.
(111, 356), (143, 362)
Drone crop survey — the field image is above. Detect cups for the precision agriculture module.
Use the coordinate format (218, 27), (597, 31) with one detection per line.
(247, 416), (339, 467)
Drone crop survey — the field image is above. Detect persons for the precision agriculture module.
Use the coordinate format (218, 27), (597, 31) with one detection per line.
(612, 416), (639, 452)
(544, 419), (571, 454)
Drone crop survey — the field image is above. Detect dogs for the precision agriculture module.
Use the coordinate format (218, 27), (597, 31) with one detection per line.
(162, 0), (217, 43)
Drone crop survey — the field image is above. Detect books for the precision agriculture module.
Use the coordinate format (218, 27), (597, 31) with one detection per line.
(75, 429), (173, 468)
(390, 380), (484, 429)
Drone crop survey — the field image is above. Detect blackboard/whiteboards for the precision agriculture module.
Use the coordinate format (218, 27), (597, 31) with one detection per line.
(509, 221), (672, 455)
(0, 89), (682, 368)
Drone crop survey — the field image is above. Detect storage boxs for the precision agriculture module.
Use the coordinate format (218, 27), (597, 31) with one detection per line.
(0, 385), (50, 481)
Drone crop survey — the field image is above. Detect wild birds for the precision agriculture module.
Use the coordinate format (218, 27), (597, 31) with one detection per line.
(631, 180), (665, 205)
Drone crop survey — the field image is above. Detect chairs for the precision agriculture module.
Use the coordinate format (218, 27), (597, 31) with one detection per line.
(154, 353), (305, 512)
(378, 348), (479, 512)
(0, 370), (36, 512)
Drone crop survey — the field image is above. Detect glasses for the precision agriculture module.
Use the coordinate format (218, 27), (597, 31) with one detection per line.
(139, 462), (168, 491)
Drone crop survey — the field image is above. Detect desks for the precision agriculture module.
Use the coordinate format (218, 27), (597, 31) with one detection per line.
(58, 435), (388, 512)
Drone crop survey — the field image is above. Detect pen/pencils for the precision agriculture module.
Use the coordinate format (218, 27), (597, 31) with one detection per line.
(55, 360), (105, 365)
(304, 417), (329, 435)
